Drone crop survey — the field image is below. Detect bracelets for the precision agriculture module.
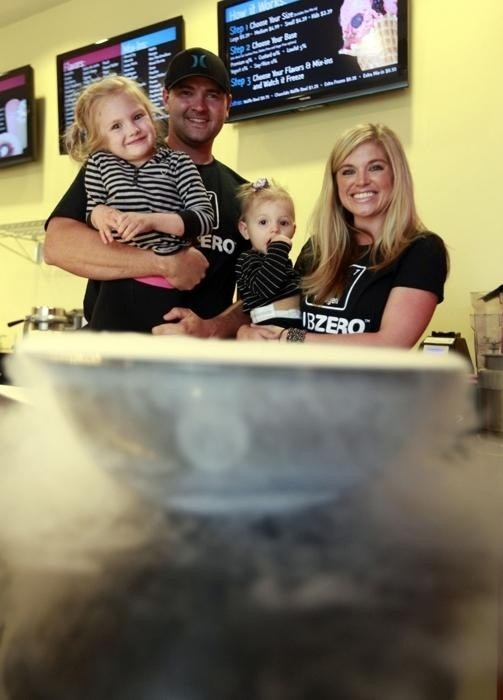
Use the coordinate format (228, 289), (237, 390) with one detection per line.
(285, 324), (308, 344)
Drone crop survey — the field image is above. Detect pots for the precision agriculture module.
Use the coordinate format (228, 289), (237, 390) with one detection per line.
(19, 306), (83, 334)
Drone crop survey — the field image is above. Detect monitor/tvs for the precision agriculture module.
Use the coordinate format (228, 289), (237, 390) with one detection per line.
(57, 15), (185, 155)
(217, 0), (409, 123)
(0, 65), (36, 169)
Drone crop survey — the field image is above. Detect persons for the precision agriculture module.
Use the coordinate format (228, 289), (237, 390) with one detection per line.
(233, 177), (304, 331)
(41, 48), (251, 339)
(231, 121), (448, 351)
(57, 72), (214, 255)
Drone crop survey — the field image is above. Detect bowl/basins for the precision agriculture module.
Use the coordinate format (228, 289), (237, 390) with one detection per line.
(21, 323), (471, 514)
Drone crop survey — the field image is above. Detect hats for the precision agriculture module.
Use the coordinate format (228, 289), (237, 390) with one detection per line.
(165, 49), (231, 93)
